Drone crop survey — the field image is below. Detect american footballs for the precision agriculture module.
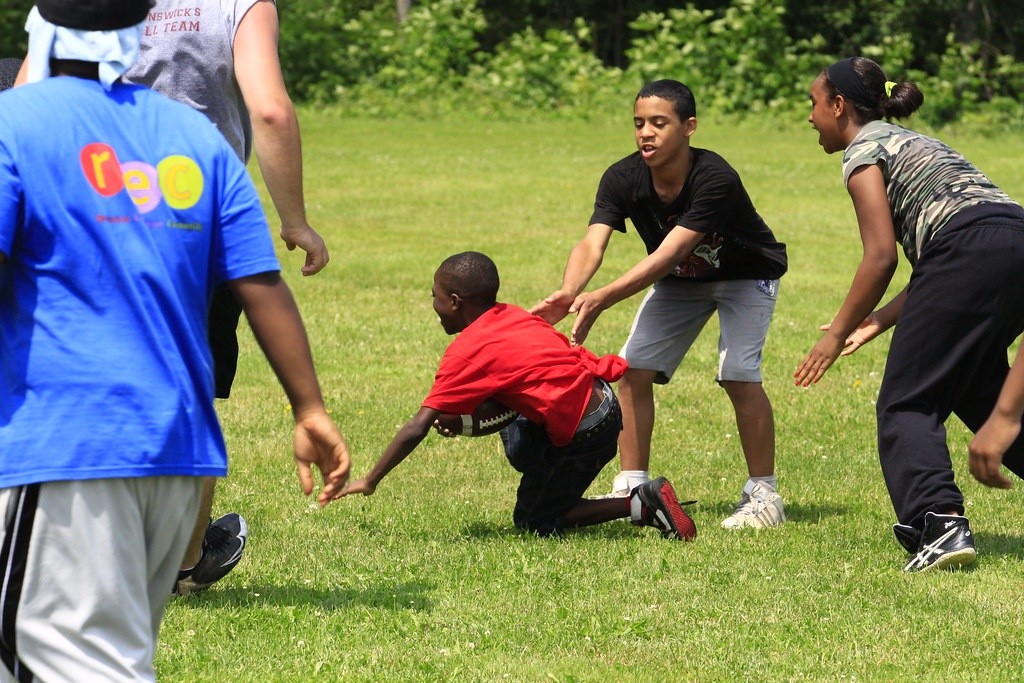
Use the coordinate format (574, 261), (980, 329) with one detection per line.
(436, 398), (520, 437)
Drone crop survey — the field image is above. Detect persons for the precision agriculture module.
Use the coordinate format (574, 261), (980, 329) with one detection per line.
(527, 78), (789, 532)
(793, 57), (1023, 573)
(1, 0), (351, 683)
(330, 248), (699, 544)
(14, 0), (333, 600)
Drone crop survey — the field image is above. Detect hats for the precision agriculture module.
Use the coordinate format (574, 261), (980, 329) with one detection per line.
(35, 0), (151, 32)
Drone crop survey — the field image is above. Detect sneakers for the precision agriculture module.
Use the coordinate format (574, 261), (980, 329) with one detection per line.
(172, 512), (247, 596)
(903, 512), (978, 574)
(628, 476), (699, 544)
(717, 478), (786, 530)
(590, 491), (636, 521)
